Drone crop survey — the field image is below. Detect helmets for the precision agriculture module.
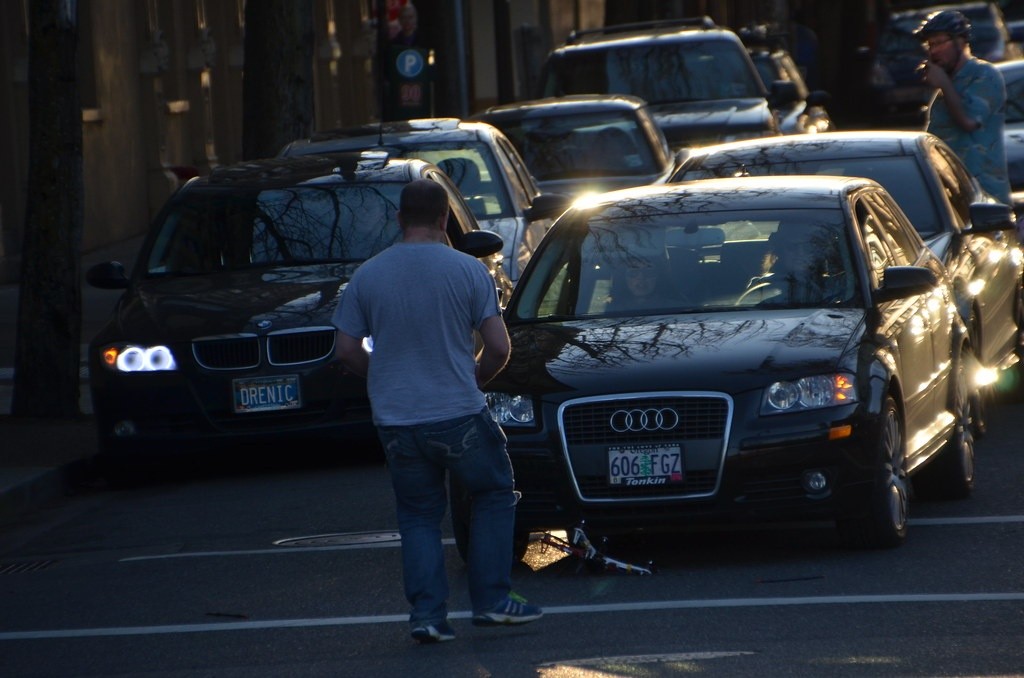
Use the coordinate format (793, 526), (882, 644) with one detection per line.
(911, 10), (972, 40)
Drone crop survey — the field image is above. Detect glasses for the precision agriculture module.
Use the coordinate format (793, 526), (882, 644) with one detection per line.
(776, 241), (821, 252)
(923, 37), (958, 52)
(626, 267), (659, 279)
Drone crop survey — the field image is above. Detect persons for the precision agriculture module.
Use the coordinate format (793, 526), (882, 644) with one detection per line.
(331, 180), (544, 642)
(913, 9), (1014, 207)
(604, 254), (683, 311)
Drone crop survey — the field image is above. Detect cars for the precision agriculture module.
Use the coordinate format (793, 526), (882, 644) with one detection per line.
(472, 93), (671, 223)
(84, 150), (513, 480)
(662, 127), (1024, 392)
(450, 173), (980, 565)
(273, 115), (573, 288)
(748, 0), (1024, 227)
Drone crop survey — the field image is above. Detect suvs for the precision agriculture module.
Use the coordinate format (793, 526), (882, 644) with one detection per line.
(530, 13), (802, 150)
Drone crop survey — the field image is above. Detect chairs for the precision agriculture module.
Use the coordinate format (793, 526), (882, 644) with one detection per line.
(593, 127), (637, 173)
(613, 245), (669, 299)
(436, 158), (486, 218)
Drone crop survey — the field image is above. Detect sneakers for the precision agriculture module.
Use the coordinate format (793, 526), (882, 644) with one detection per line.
(471, 592), (543, 625)
(410, 620), (460, 642)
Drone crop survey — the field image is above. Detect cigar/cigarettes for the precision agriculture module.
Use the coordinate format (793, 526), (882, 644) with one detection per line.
(916, 58), (937, 71)
